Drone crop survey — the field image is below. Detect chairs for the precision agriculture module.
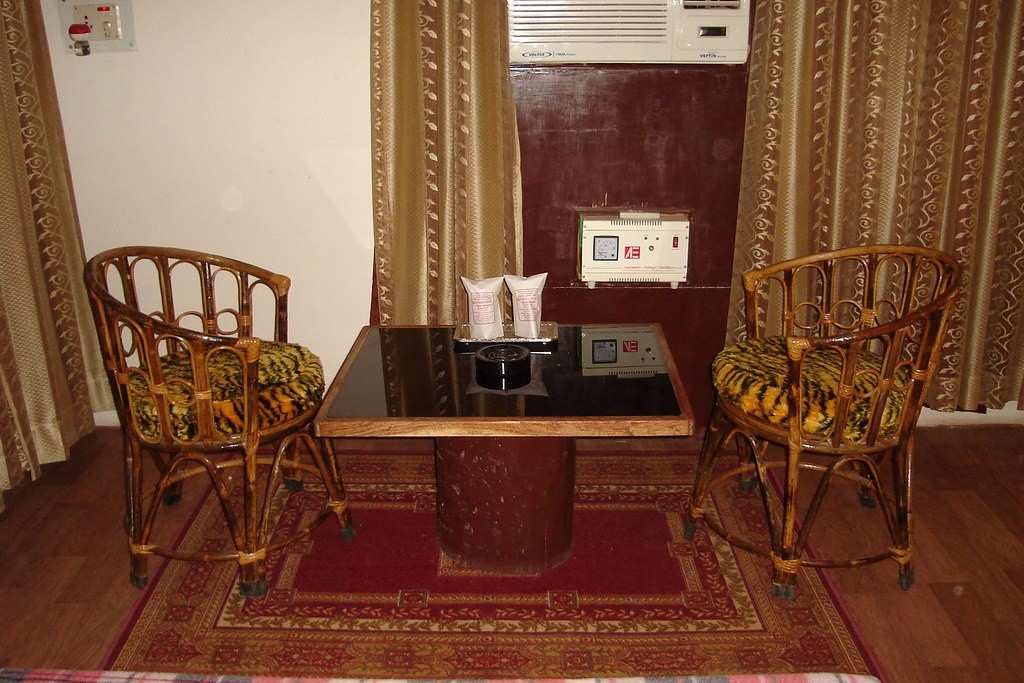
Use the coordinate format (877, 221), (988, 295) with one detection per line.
(82, 246), (357, 601)
(685, 244), (964, 603)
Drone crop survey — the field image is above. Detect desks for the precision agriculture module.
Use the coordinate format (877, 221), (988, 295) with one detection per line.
(311, 322), (696, 575)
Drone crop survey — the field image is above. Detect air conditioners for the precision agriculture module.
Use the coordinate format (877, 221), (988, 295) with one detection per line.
(507, 0), (750, 65)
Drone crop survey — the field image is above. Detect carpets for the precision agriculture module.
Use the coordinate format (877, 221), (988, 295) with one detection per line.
(97, 448), (893, 683)
